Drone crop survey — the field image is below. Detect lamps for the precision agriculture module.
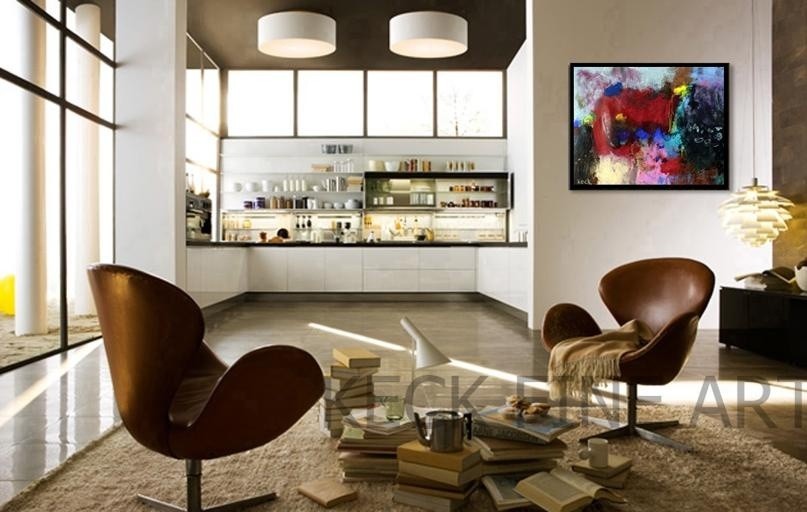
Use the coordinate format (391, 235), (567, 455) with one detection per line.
(257, 12), (336, 59)
(401, 318), (452, 413)
(715, 1), (795, 249)
(388, 10), (468, 59)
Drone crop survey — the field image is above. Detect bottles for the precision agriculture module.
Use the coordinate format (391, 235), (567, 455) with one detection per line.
(222, 214), (430, 243)
(243, 195), (318, 210)
(441, 198), (498, 207)
(333, 158), (355, 173)
(449, 184), (494, 192)
(400, 159), (476, 173)
(186, 173), (196, 193)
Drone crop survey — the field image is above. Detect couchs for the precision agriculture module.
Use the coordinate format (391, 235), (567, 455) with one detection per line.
(720, 287), (807, 369)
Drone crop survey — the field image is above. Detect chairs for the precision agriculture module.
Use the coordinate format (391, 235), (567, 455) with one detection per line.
(543, 256), (714, 452)
(86, 264), (325, 511)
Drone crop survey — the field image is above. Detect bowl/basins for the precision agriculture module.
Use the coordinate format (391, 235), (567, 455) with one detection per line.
(498, 402), (549, 424)
(324, 199), (361, 210)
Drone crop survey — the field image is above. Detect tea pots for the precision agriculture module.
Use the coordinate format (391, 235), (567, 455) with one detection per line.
(414, 410), (472, 453)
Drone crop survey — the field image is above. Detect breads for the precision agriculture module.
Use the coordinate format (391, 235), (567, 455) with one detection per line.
(498, 394), (551, 423)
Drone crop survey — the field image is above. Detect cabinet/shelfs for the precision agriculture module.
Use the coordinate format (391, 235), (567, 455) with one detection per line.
(363, 169), (514, 241)
(221, 172), (363, 244)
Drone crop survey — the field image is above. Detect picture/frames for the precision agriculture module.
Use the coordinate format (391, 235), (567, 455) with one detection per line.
(569, 61), (732, 191)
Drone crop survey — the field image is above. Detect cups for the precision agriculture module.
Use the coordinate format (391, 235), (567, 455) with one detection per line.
(326, 179), (335, 192)
(321, 144), (353, 153)
(370, 159), (400, 172)
(385, 395), (405, 420)
(283, 179), (308, 192)
(577, 438), (609, 469)
(261, 179), (274, 192)
(335, 176), (345, 191)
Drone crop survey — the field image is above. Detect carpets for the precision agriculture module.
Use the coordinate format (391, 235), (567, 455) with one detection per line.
(2, 402), (807, 512)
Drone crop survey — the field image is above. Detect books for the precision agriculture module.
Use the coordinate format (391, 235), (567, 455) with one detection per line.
(298, 343), (634, 512)
